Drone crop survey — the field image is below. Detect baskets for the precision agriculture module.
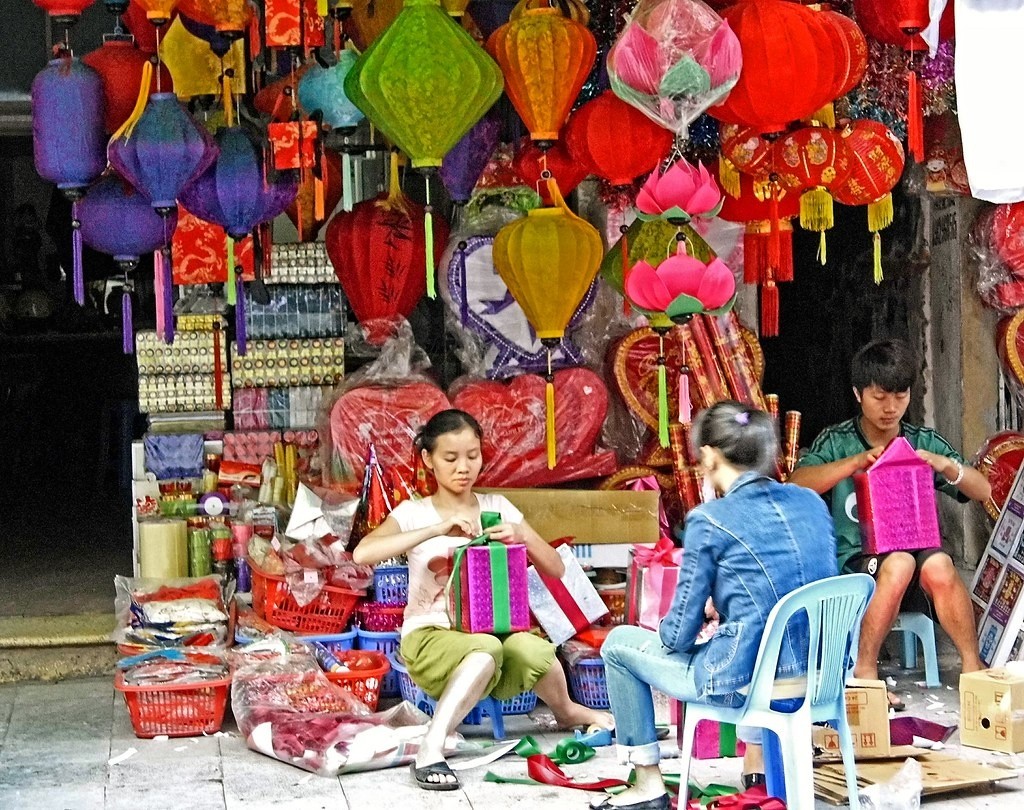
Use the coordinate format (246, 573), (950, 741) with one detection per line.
(111, 554), (625, 736)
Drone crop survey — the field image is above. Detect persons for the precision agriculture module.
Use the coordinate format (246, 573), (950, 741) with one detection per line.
(353, 409), (615, 791)
(784, 338), (992, 710)
(587, 400), (854, 810)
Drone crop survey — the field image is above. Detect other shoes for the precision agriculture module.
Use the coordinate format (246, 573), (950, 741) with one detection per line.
(741, 772), (765, 789)
(590, 792), (672, 810)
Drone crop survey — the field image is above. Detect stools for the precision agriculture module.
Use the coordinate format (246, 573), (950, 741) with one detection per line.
(415, 686), (507, 740)
(851, 612), (942, 687)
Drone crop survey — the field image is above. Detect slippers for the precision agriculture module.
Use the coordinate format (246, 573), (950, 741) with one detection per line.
(410, 762), (459, 790)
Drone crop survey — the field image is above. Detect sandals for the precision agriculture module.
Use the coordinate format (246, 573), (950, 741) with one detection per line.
(887, 689), (906, 710)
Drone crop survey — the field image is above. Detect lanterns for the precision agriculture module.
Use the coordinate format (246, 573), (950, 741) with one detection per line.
(32, 0), (357, 409)
(338, 0), (904, 470)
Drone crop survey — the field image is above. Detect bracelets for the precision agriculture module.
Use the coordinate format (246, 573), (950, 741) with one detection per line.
(942, 458), (964, 485)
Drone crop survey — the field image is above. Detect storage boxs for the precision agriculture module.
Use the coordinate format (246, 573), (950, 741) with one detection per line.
(959, 667), (1024, 754)
(115, 486), (890, 758)
(968, 458), (1024, 668)
(852, 437), (941, 554)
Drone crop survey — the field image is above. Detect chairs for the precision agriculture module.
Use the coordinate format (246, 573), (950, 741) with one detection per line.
(676, 573), (876, 810)
